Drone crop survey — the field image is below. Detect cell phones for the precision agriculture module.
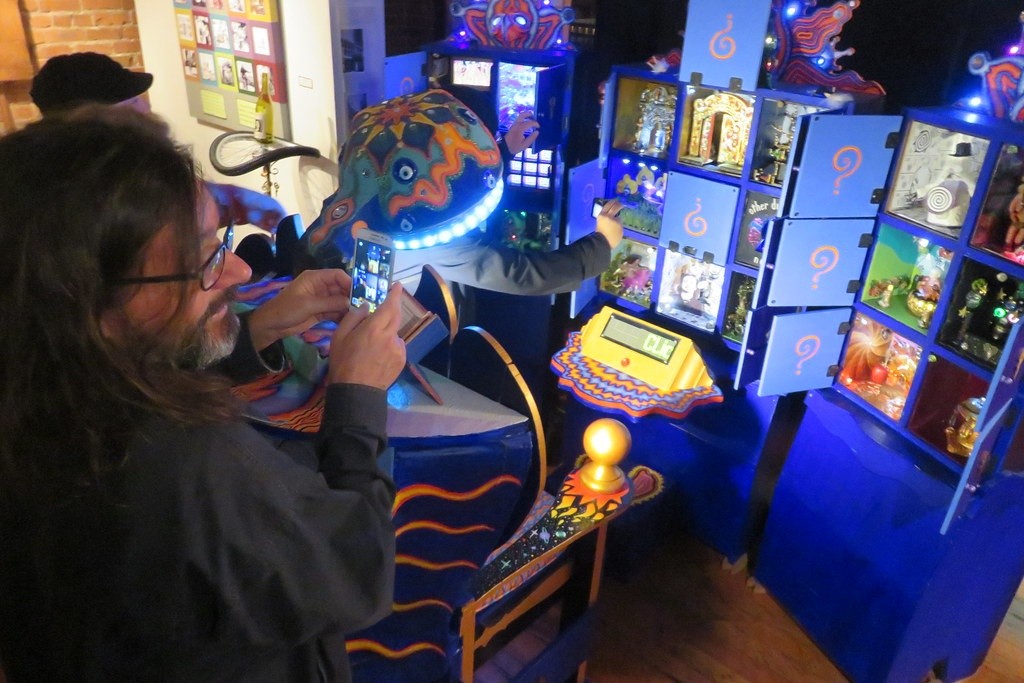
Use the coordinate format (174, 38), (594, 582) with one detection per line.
(347, 227), (397, 317)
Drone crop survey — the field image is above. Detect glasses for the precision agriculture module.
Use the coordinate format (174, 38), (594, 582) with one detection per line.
(112, 215), (235, 291)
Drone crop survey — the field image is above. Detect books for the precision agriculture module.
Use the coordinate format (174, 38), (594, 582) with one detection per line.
(385, 281), (451, 365)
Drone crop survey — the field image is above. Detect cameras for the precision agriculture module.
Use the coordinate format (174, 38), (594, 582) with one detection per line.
(591, 198), (620, 219)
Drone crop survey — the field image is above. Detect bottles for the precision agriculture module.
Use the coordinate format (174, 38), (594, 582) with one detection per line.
(253, 73), (274, 143)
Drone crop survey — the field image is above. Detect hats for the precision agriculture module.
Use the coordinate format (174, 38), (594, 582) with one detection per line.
(29, 52), (153, 118)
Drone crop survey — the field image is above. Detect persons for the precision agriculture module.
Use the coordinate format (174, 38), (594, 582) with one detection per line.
(0, 112), (410, 683)
(29, 52), (153, 119)
(391, 108), (630, 301)
